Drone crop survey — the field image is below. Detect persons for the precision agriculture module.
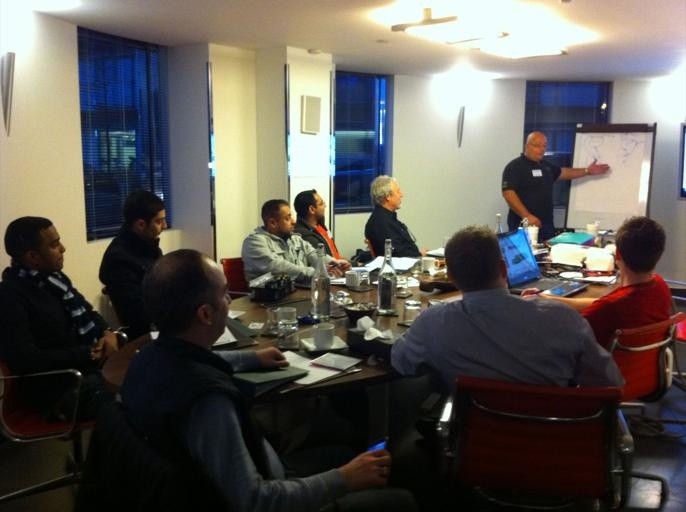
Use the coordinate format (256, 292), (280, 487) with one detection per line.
(293, 187), (362, 277)
(99, 190), (169, 336)
(500, 130), (612, 234)
(0, 214), (143, 419)
(520, 213), (675, 354)
(387, 223), (626, 402)
(120, 249), (426, 511)
(240, 196), (353, 284)
(365, 173), (433, 257)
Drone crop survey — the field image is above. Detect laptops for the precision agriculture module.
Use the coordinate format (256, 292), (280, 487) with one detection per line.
(497, 230), (589, 297)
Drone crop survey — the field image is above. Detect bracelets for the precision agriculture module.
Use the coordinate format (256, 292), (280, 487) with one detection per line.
(583, 166), (589, 176)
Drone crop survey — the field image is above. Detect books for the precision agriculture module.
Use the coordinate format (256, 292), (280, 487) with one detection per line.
(425, 246), (447, 259)
(309, 350), (364, 372)
(547, 229), (598, 245)
(353, 254), (420, 274)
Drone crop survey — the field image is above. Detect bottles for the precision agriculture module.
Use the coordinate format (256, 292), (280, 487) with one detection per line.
(521, 216), (531, 247)
(494, 212), (504, 234)
(374, 242), (401, 316)
(308, 243), (334, 320)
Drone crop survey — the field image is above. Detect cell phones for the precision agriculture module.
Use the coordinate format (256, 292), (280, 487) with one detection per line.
(367, 435), (389, 451)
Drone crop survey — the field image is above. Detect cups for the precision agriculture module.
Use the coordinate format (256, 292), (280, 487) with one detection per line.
(527, 226), (542, 246)
(263, 254), (440, 353)
(586, 225), (598, 239)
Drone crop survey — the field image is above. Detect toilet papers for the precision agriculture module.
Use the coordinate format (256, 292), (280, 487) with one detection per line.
(518, 227), (540, 243)
(587, 224), (598, 235)
(585, 246), (615, 271)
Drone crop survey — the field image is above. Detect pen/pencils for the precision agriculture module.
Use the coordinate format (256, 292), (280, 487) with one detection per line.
(328, 264), (337, 270)
(528, 289), (544, 295)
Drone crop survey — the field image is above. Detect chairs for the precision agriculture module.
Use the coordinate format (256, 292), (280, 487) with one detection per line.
(609, 311), (686, 446)
(364, 238), (375, 258)
(0, 473), (88, 512)
(668, 286), (686, 390)
(217, 255), (253, 304)
(409, 370), (637, 511)
(0, 355), (91, 469)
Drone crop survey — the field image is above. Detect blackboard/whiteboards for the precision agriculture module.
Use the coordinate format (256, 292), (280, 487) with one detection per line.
(564, 122), (657, 232)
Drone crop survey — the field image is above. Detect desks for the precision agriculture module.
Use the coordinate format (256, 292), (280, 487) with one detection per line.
(101, 255), (616, 512)
